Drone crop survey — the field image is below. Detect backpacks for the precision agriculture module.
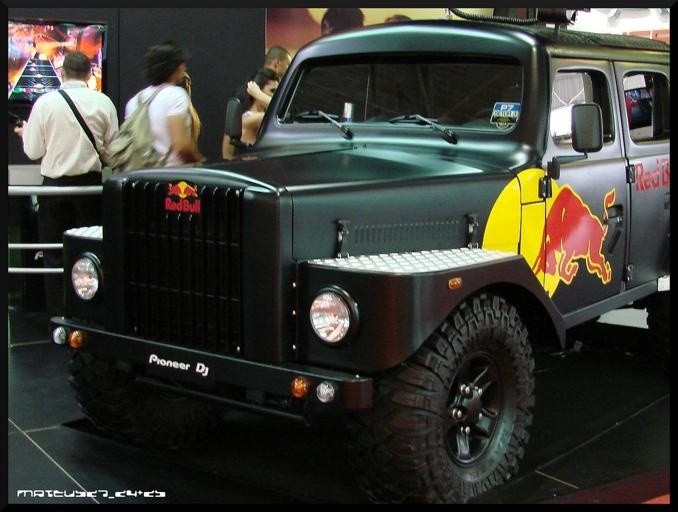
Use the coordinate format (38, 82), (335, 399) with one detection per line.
(105, 84), (174, 177)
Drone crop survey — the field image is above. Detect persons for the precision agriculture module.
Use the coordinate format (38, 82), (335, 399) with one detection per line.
(124, 41), (208, 167)
(12, 51), (120, 346)
(221, 67), (282, 161)
(231, 45), (292, 111)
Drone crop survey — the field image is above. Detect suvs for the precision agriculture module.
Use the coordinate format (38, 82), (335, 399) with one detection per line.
(48, 18), (669, 504)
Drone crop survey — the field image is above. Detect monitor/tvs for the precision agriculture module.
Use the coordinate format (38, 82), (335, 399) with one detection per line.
(7, 17), (108, 109)
